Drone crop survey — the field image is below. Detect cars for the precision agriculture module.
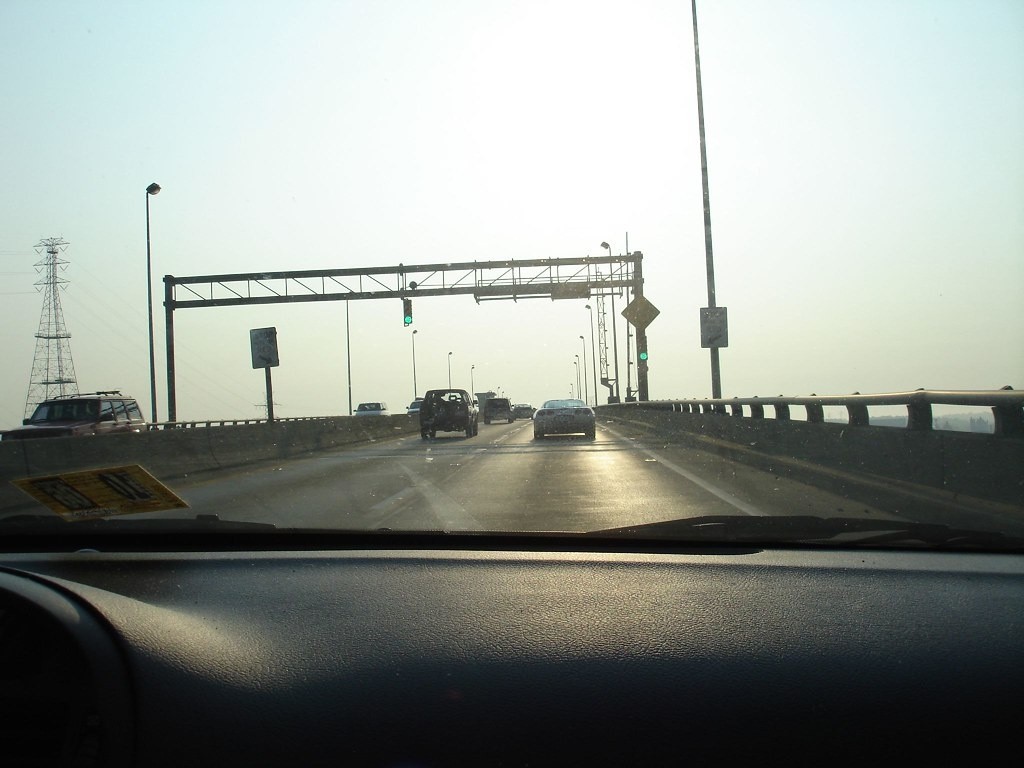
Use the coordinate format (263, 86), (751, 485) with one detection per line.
(533, 399), (595, 440)
(405, 400), (424, 413)
(515, 404), (533, 420)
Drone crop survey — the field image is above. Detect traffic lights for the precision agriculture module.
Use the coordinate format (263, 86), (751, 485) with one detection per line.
(640, 336), (648, 361)
(402, 299), (412, 324)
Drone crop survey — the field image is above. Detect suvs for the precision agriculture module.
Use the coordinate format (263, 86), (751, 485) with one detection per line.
(483, 398), (513, 424)
(12, 391), (146, 436)
(419, 387), (479, 440)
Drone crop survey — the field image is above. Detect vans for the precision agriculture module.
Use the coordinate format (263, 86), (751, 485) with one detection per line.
(353, 402), (390, 416)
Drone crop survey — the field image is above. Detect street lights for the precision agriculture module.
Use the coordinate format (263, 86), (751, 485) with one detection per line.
(471, 367), (474, 401)
(579, 336), (587, 405)
(497, 387), (500, 398)
(585, 305), (597, 405)
(502, 391), (504, 397)
(601, 241), (620, 396)
(574, 362), (579, 399)
(569, 383), (573, 399)
(145, 182), (160, 423)
(412, 330), (418, 399)
(448, 352), (452, 388)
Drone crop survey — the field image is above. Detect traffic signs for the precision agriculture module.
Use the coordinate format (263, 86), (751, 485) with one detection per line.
(249, 326), (279, 369)
(700, 306), (728, 348)
